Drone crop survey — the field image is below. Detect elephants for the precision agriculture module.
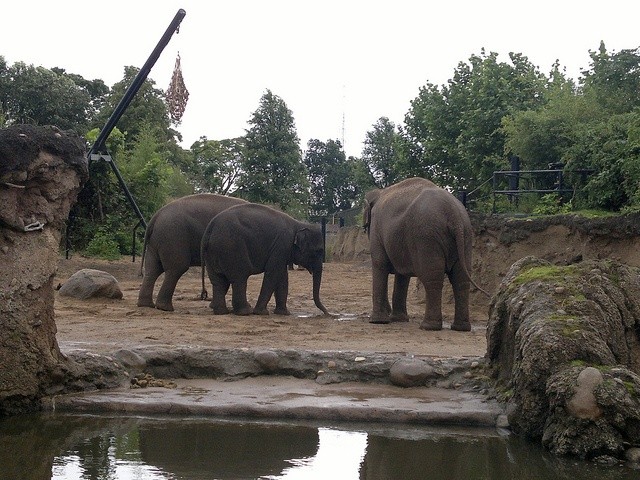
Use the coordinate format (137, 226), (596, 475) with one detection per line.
(199, 202), (334, 317)
(135, 192), (256, 312)
(362, 176), (493, 332)
